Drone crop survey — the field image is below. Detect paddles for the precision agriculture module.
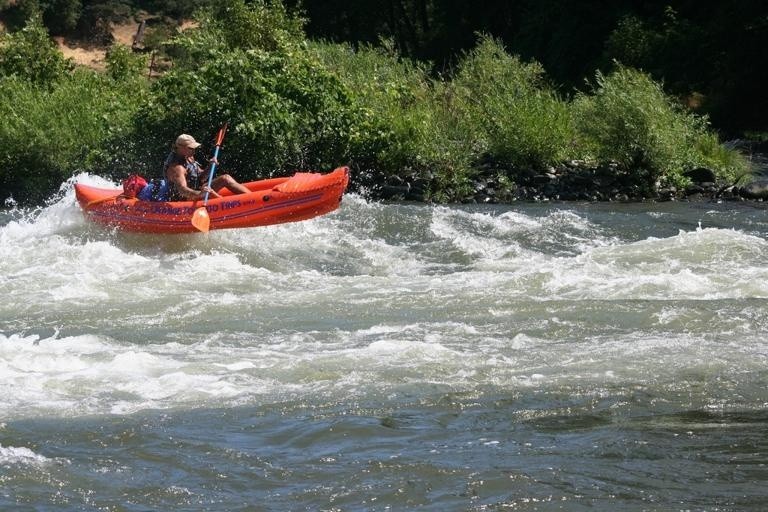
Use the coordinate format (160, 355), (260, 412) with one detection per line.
(191, 123), (227, 232)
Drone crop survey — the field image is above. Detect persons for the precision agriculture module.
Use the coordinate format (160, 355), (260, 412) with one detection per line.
(162, 134), (252, 202)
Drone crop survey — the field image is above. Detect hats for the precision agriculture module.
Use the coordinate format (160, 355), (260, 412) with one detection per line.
(175, 134), (201, 149)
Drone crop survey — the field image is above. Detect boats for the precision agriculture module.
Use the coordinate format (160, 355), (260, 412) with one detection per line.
(73, 166), (349, 234)
